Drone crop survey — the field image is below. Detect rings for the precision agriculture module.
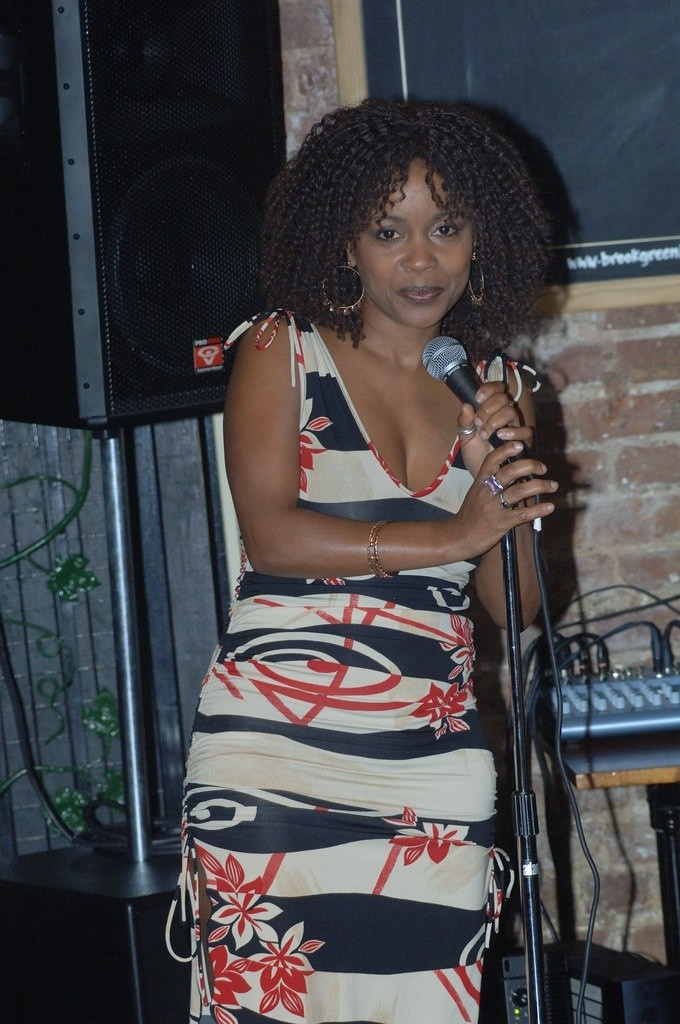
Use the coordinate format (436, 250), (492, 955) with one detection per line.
(457, 424), (476, 434)
(484, 476), (504, 495)
(499, 494), (510, 507)
(509, 401), (515, 407)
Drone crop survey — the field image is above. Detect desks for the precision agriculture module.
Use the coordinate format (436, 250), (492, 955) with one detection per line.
(525, 722), (680, 977)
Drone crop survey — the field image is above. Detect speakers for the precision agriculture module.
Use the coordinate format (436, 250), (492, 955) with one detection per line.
(0, 0), (286, 429)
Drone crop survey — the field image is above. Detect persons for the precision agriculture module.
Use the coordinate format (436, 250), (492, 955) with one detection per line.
(167, 100), (558, 1023)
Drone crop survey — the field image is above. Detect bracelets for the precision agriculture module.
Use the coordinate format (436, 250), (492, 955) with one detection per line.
(366, 520), (398, 579)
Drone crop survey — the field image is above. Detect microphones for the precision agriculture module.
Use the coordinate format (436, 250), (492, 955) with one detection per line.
(422, 336), (535, 480)
